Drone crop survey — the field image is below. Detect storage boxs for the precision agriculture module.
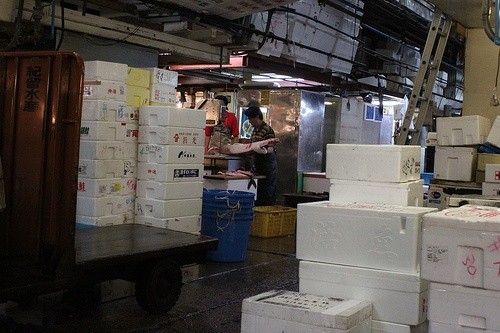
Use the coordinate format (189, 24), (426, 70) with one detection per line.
(76, 0), (500, 333)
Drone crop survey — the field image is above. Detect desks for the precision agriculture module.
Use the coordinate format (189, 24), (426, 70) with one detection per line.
(205, 154), (242, 175)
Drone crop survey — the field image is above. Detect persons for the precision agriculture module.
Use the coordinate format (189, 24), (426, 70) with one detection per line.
(245, 106), (280, 206)
(203, 94), (240, 156)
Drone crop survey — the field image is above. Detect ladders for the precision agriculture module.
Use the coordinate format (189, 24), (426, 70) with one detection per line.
(396, 6), (453, 146)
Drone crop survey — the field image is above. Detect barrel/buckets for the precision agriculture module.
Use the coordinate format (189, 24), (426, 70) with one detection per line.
(201, 189), (255, 262)
